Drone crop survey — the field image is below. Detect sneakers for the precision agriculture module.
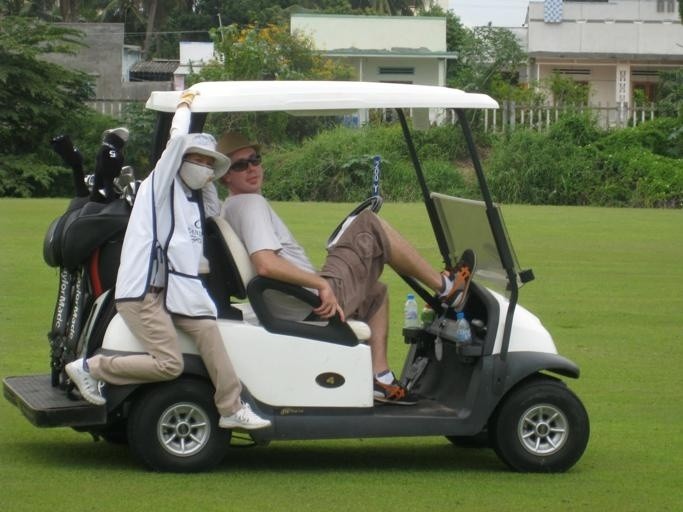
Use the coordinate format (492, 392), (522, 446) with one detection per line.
(219, 403), (271, 430)
(440, 248), (477, 313)
(374, 378), (418, 405)
(65, 357), (107, 404)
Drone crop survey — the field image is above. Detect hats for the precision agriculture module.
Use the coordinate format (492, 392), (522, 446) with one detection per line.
(182, 133), (231, 179)
(219, 133), (263, 155)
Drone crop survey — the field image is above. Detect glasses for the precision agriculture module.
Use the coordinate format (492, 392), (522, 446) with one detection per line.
(230, 155), (262, 172)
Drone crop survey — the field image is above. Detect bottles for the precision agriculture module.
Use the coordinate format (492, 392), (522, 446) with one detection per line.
(455, 312), (476, 364)
(403, 294), (418, 331)
(420, 302), (437, 333)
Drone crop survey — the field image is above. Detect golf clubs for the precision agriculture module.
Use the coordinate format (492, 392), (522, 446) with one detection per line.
(83, 165), (142, 211)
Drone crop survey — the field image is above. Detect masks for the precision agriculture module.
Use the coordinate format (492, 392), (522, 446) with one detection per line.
(180, 159), (215, 189)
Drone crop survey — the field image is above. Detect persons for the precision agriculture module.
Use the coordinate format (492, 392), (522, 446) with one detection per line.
(63, 87), (271, 431)
(214, 131), (476, 405)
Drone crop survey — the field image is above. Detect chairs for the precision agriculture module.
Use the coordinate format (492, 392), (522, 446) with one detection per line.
(206, 215), (371, 346)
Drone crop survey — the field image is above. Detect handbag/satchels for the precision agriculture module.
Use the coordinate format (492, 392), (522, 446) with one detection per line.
(43, 197), (130, 267)
